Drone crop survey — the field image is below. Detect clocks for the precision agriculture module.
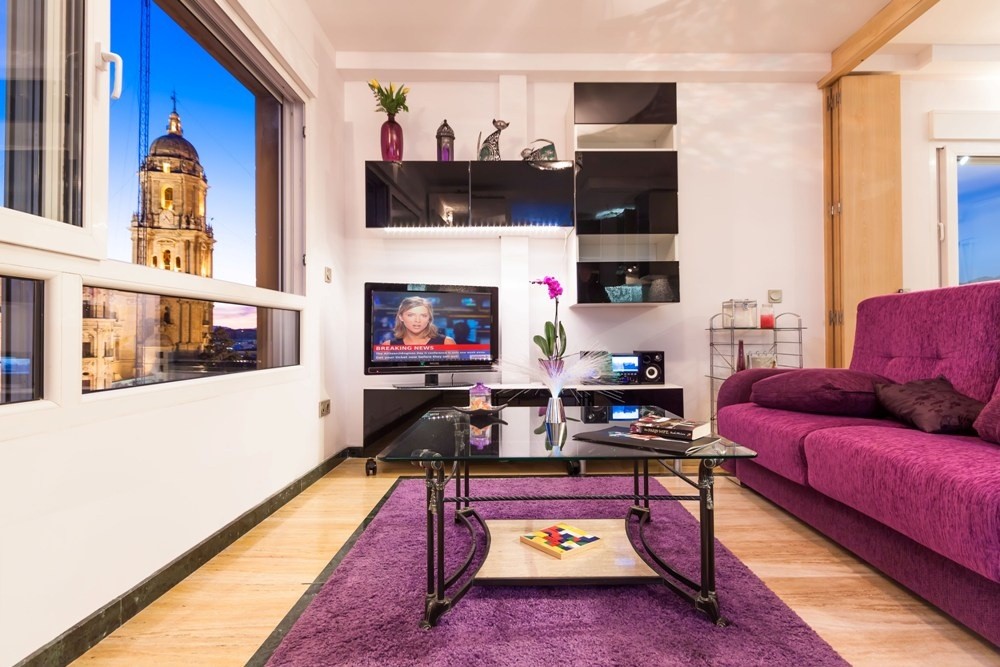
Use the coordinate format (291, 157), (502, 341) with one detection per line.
(158, 210), (174, 225)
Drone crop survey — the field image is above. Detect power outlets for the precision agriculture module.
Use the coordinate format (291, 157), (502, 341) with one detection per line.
(319, 399), (331, 418)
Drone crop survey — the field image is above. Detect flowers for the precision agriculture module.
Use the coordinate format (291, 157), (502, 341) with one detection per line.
(526, 273), (568, 359)
(365, 77), (413, 114)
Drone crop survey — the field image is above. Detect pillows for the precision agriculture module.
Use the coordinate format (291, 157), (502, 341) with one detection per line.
(972, 394), (1000, 444)
(748, 366), (895, 416)
(872, 373), (988, 436)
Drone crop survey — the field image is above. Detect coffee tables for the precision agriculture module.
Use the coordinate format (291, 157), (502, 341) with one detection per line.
(376, 405), (760, 630)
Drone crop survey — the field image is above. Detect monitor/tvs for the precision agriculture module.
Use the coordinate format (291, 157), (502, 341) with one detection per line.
(363, 282), (499, 388)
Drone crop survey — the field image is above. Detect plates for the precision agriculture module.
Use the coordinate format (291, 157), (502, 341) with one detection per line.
(453, 403), (508, 413)
(452, 416), (508, 428)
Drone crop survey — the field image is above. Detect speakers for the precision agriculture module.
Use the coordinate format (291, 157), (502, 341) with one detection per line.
(633, 351), (664, 385)
(579, 351), (612, 384)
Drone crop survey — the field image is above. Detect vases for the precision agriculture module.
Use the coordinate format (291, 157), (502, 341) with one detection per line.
(539, 358), (565, 379)
(380, 114), (404, 162)
(543, 397), (570, 453)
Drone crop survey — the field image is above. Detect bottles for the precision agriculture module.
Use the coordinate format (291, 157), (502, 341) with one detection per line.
(737, 340), (746, 372)
(760, 303), (775, 328)
(469, 424), (492, 450)
(469, 382), (491, 410)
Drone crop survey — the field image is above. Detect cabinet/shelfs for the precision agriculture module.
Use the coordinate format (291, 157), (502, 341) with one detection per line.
(361, 158), (574, 241)
(565, 80), (686, 309)
(704, 312), (808, 433)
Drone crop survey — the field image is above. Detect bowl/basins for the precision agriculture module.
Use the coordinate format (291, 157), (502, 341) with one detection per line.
(605, 286), (643, 303)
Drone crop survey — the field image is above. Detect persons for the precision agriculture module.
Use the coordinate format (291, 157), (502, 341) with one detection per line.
(383, 297), (457, 346)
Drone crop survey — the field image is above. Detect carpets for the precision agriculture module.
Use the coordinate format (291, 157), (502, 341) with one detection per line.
(241, 472), (857, 667)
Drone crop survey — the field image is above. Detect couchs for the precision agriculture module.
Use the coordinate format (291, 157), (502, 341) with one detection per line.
(715, 276), (1000, 651)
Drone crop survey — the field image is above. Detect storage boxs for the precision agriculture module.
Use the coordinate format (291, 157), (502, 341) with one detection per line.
(721, 297), (759, 330)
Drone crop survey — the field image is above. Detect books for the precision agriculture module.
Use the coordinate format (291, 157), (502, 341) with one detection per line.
(630, 414), (711, 440)
(573, 425), (719, 456)
